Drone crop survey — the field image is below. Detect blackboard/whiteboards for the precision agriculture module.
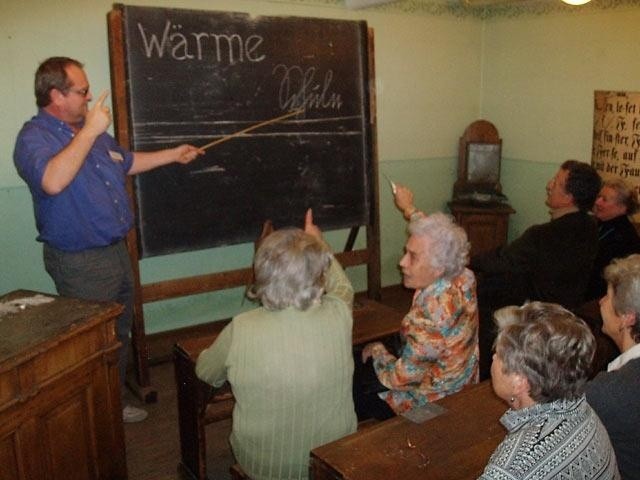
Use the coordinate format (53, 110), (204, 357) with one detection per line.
(106, 4), (381, 261)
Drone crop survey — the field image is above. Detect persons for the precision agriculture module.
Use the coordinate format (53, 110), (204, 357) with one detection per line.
(469, 160), (602, 378)
(194, 207), (359, 480)
(596, 176), (639, 258)
(14, 57), (205, 424)
(475, 301), (621, 480)
(581, 253), (639, 479)
(362, 181), (480, 423)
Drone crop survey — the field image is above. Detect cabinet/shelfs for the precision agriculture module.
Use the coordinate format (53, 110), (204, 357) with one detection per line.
(446, 200), (516, 258)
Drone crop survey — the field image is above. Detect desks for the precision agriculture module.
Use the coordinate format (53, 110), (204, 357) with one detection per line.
(0, 288), (128, 480)
(173, 295), (406, 480)
(307, 337), (621, 480)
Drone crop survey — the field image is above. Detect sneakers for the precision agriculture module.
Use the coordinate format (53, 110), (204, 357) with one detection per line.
(123, 406), (148, 423)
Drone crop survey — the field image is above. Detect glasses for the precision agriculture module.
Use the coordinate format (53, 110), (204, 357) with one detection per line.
(70, 89), (89, 95)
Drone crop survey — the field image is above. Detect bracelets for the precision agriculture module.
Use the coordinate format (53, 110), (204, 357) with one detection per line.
(318, 240), (328, 249)
(369, 344), (386, 353)
